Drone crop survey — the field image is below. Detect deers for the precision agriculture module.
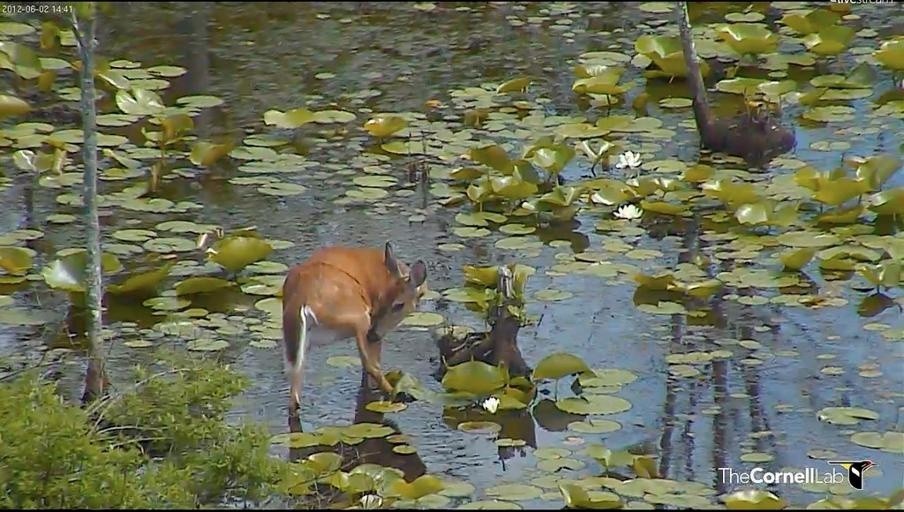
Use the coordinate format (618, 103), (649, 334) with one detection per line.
(277, 240), (430, 421)
